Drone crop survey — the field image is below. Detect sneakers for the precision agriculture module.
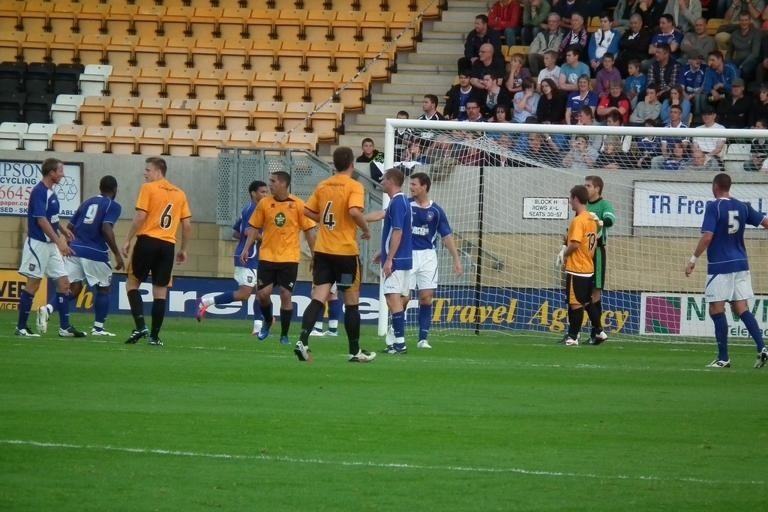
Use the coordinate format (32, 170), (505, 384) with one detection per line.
(705, 358), (730, 368)
(14, 306), (164, 347)
(754, 347), (768, 368)
(293, 340), (309, 359)
(252, 329), (337, 344)
(582, 332), (607, 346)
(558, 330), (580, 346)
(383, 325), (432, 354)
(348, 349), (376, 363)
(194, 297), (208, 321)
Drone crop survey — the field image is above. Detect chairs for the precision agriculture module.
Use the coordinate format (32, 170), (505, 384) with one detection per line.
(449, 13), (729, 85)
(0, 1), (446, 156)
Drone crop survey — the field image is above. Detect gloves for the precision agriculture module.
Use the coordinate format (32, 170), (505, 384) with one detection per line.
(556, 244), (566, 267)
(590, 211), (604, 227)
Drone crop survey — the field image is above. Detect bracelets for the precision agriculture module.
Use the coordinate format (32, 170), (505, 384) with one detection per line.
(690, 255), (698, 264)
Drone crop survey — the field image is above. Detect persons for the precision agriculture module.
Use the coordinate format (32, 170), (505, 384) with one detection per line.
(392, 2), (767, 170)
(197, 180), (269, 337)
(563, 175), (616, 344)
(356, 137), (384, 162)
(238, 170), (317, 344)
(293, 147), (377, 363)
(373, 168), (413, 356)
(121, 157), (193, 346)
(14, 158), (86, 338)
(36, 176), (126, 340)
(555, 184), (607, 347)
(684, 174), (767, 369)
(363, 172), (465, 350)
